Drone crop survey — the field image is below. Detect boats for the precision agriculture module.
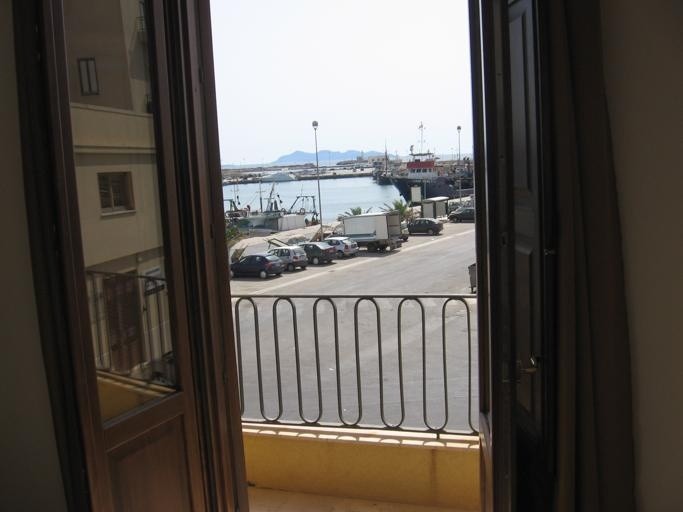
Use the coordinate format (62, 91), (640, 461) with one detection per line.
(223, 171), (319, 235)
(371, 122), (473, 203)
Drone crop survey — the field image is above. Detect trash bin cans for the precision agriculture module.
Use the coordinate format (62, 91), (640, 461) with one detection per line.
(468, 263), (476, 293)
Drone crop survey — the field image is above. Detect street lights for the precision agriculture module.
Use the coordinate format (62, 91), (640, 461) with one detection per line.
(312, 121), (324, 241)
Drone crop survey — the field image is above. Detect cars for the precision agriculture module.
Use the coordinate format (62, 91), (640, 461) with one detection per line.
(401, 218), (443, 241)
(230, 236), (359, 279)
(447, 194), (476, 222)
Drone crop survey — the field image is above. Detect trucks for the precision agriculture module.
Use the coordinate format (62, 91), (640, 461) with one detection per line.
(332, 211), (402, 253)
